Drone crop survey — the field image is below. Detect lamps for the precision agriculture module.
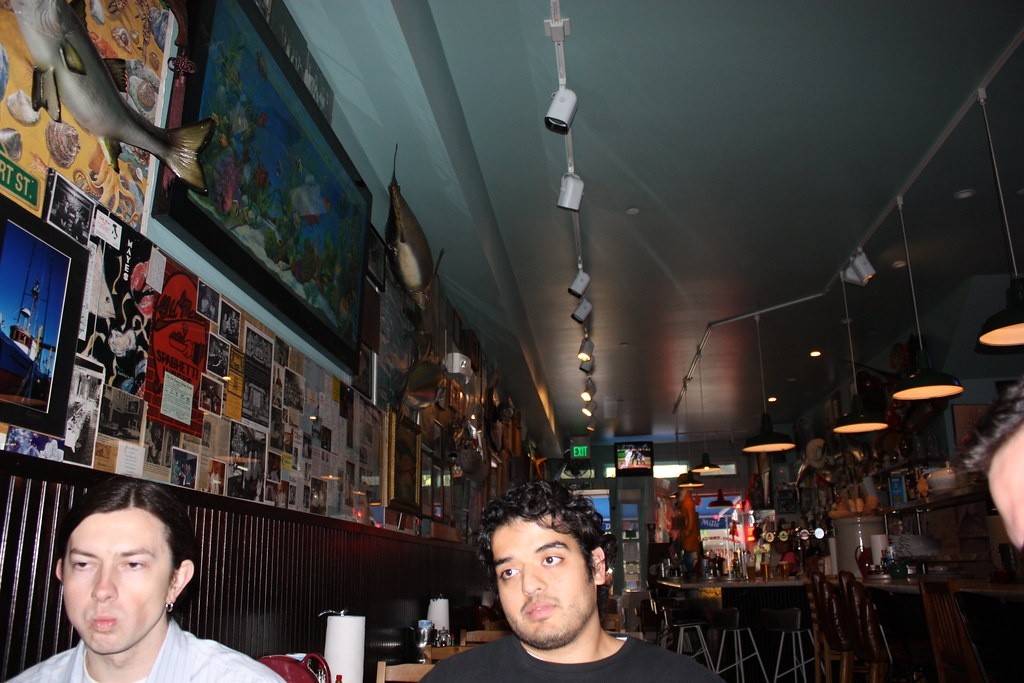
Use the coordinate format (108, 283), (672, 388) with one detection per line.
(890, 194), (964, 402)
(844, 247), (876, 290)
(977, 84), (1024, 346)
(832, 283), (888, 434)
(558, 171), (583, 213)
(570, 268), (598, 431)
(674, 355), (736, 508)
(546, 88), (578, 131)
(742, 315), (795, 453)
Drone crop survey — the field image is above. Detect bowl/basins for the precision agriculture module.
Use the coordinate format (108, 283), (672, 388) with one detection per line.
(927, 461), (968, 488)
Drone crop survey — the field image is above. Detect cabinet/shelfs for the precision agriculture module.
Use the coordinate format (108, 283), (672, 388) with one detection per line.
(871, 456), (943, 514)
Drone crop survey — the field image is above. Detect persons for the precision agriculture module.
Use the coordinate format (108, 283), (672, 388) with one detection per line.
(6, 479), (287, 683)
(54, 200), (82, 241)
(957, 379), (1024, 560)
(419, 479), (726, 683)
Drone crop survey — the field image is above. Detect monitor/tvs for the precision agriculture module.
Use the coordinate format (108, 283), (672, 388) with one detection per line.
(614, 441), (654, 478)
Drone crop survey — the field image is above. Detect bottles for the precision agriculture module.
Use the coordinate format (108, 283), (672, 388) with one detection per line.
(869, 564), (877, 575)
(881, 550), (889, 574)
(430, 624), (455, 648)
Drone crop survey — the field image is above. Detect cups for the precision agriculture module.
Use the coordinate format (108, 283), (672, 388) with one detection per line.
(745, 562), (757, 581)
(761, 562), (770, 581)
(779, 561), (789, 580)
(417, 620), (432, 647)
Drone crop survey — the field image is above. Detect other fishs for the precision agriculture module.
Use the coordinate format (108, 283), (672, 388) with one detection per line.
(10, 0), (215, 195)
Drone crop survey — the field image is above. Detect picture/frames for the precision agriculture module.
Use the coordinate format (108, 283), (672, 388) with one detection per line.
(0, 191), (91, 439)
(152, 1), (521, 537)
(951, 404), (989, 450)
(755, 450), (774, 511)
(778, 498), (798, 514)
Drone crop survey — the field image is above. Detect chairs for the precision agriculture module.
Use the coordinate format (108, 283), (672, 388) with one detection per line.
(811, 570), (1024, 683)
(376, 613), (624, 683)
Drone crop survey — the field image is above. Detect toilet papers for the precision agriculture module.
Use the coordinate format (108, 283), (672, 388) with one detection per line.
(481, 590), (495, 607)
(427, 598), (450, 636)
(323, 615), (366, 683)
(869, 534), (889, 565)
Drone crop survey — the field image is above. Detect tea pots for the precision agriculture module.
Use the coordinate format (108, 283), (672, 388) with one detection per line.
(887, 558), (908, 578)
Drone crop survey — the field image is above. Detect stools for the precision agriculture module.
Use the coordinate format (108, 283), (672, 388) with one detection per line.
(637, 599), (826, 683)
